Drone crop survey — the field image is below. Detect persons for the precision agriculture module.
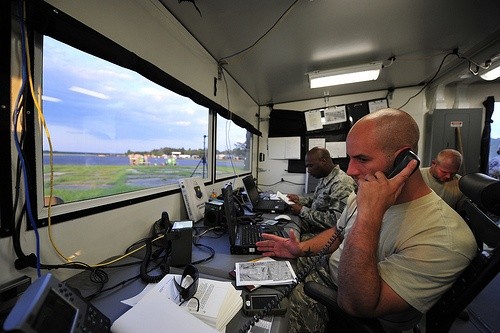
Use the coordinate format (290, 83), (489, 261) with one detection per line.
(287, 146), (356, 274)
(419, 149), (464, 208)
(489, 145), (500, 179)
(255, 108), (477, 333)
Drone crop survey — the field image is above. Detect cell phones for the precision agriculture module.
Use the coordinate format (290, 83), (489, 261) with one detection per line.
(243, 292), (288, 315)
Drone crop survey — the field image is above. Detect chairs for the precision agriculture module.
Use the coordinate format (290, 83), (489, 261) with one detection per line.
(426, 172), (499, 333)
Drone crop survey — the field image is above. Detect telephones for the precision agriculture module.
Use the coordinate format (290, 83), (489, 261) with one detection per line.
(354, 149), (420, 195)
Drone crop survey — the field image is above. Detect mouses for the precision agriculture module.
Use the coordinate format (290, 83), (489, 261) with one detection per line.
(274, 214), (291, 221)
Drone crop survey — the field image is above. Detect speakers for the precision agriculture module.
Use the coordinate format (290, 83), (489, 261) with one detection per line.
(171, 221), (194, 266)
(204, 201), (225, 233)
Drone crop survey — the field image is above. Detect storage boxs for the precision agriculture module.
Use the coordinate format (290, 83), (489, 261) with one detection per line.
(179, 176), (209, 223)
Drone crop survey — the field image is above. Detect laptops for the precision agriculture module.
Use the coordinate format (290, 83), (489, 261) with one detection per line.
(221, 181), (290, 255)
(242, 176), (286, 213)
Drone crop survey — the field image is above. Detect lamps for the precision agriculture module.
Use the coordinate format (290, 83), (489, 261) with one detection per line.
(308, 60), (383, 89)
(477, 52), (500, 81)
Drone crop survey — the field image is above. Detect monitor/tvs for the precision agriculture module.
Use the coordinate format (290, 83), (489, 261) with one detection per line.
(3, 273), (111, 333)
